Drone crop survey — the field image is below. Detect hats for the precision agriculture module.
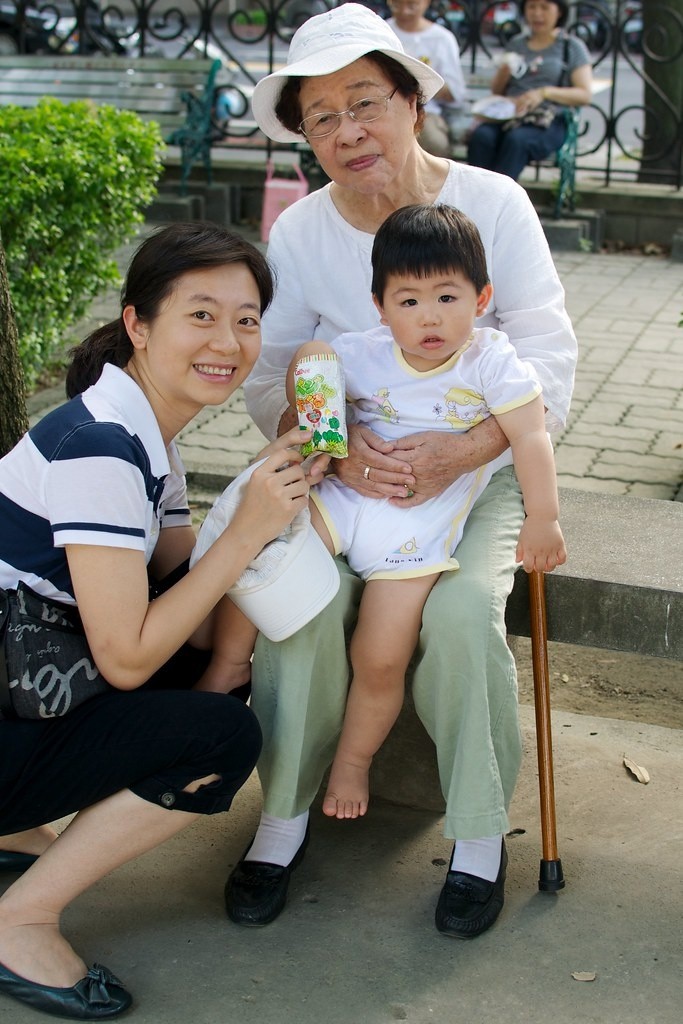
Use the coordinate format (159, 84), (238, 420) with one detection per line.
(250, 2), (444, 143)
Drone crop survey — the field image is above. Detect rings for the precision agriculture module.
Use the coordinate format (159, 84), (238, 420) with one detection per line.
(363, 466), (370, 480)
(404, 484), (414, 497)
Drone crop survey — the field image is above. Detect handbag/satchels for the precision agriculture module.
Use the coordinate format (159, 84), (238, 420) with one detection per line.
(503, 106), (556, 130)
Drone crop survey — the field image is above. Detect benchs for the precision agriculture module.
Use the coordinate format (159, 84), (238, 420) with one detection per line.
(0, 56), (222, 198)
(323, 486), (683, 813)
(295, 67), (582, 215)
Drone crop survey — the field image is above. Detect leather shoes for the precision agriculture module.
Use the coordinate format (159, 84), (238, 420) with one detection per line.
(224, 817), (310, 925)
(436, 832), (508, 938)
(0, 954), (133, 1021)
(0, 833), (60, 873)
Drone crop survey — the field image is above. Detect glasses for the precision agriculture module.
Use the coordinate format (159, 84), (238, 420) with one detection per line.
(297, 80), (408, 139)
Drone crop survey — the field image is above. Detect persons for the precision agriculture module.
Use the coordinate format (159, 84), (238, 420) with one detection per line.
(0, 220), (332, 1019)
(194, 4), (595, 943)
(197, 204), (567, 818)
(384, 0), (592, 182)
